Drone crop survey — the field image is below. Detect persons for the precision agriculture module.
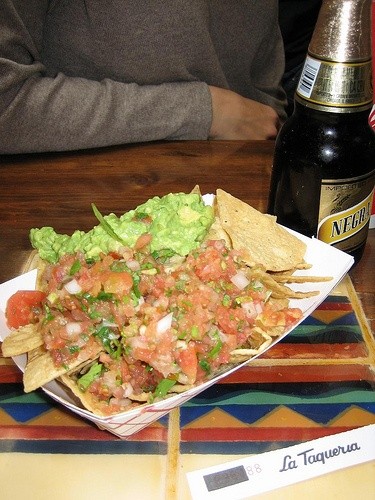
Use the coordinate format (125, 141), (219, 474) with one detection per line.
(0, 0), (314, 157)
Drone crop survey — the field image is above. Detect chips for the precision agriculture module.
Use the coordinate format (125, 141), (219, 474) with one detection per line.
(1, 315), (111, 416)
(211, 188), (333, 299)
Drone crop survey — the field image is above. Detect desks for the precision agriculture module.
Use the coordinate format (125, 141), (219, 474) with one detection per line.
(0, 138), (374, 500)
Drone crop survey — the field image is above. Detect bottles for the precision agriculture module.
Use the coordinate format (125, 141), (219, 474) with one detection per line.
(268, 0), (375, 273)
